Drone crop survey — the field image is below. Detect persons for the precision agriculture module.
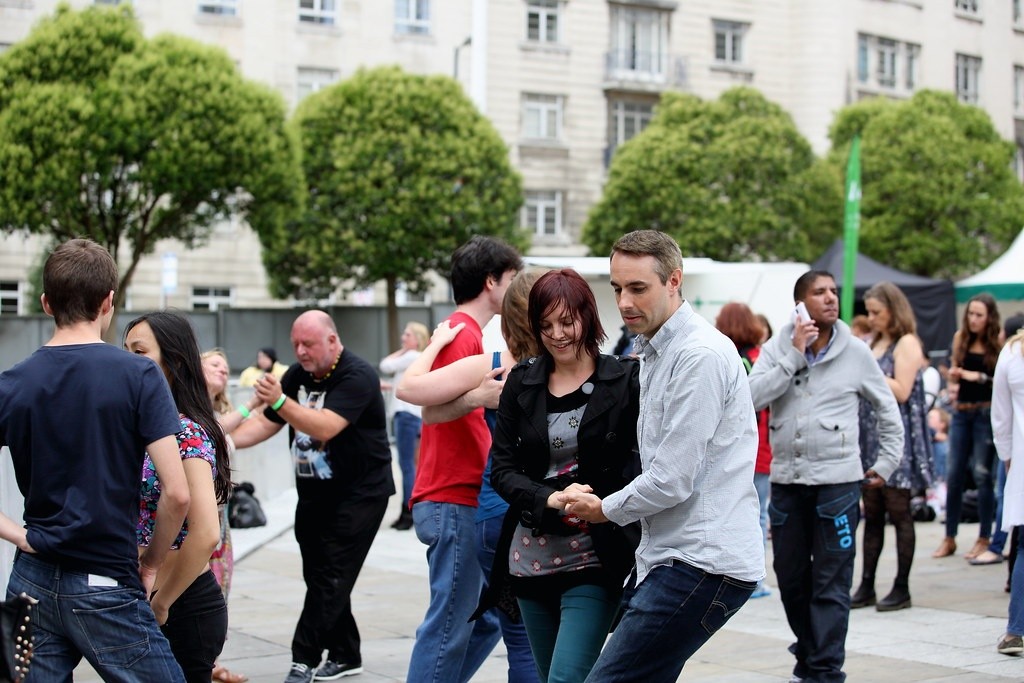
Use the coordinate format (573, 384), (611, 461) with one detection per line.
(125, 312), (228, 683)
(230, 310), (396, 683)
(241, 347), (287, 387)
(200, 348), (265, 683)
(1, 238), (190, 682)
(382, 322), (430, 530)
(933, 293), (1023, 656)
(395, 238), (554, 683)
(558, 230), (764, 683)
(489, 268), (641, 683)
(717, 269), (941, 682)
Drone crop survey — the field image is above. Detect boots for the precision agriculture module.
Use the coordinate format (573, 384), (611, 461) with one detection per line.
(877, 579), (912, 612)
(849, 580), (877, 610)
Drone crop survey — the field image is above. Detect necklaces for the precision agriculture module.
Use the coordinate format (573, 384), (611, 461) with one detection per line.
(309, 353), (340, 383)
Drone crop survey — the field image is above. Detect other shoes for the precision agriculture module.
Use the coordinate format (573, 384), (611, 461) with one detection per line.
(1005, 586), (1011, 593)
(931, 537), (956, 557)
(997, 630), (1024, 653)
(211, 667), (249, 683)
(963, 540), (991, 558)
(750, 582), (771, 598)
(969, 550), (1003, 565)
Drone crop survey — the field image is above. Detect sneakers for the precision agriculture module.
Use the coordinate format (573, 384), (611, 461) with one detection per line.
(285, 648), (329, 683)
(313, 654), (363, 681)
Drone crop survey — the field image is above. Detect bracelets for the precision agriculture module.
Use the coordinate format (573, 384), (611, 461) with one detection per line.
(272, 394), (286, 411)
(239, 405), (249, 416)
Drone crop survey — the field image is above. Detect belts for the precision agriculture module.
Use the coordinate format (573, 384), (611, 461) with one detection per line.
(952, 401), (993, 409)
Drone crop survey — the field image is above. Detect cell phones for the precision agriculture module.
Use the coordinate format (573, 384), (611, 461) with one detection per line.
(794, 302), (818, 348)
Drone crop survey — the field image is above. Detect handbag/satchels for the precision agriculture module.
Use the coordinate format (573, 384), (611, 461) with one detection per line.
(228, 482), (269, 528)
(523, 469), (591, 538)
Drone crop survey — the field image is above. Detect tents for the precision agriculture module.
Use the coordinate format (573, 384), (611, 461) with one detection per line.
(954, 228), (1024, 332)
(813, 237), (954, 352)
